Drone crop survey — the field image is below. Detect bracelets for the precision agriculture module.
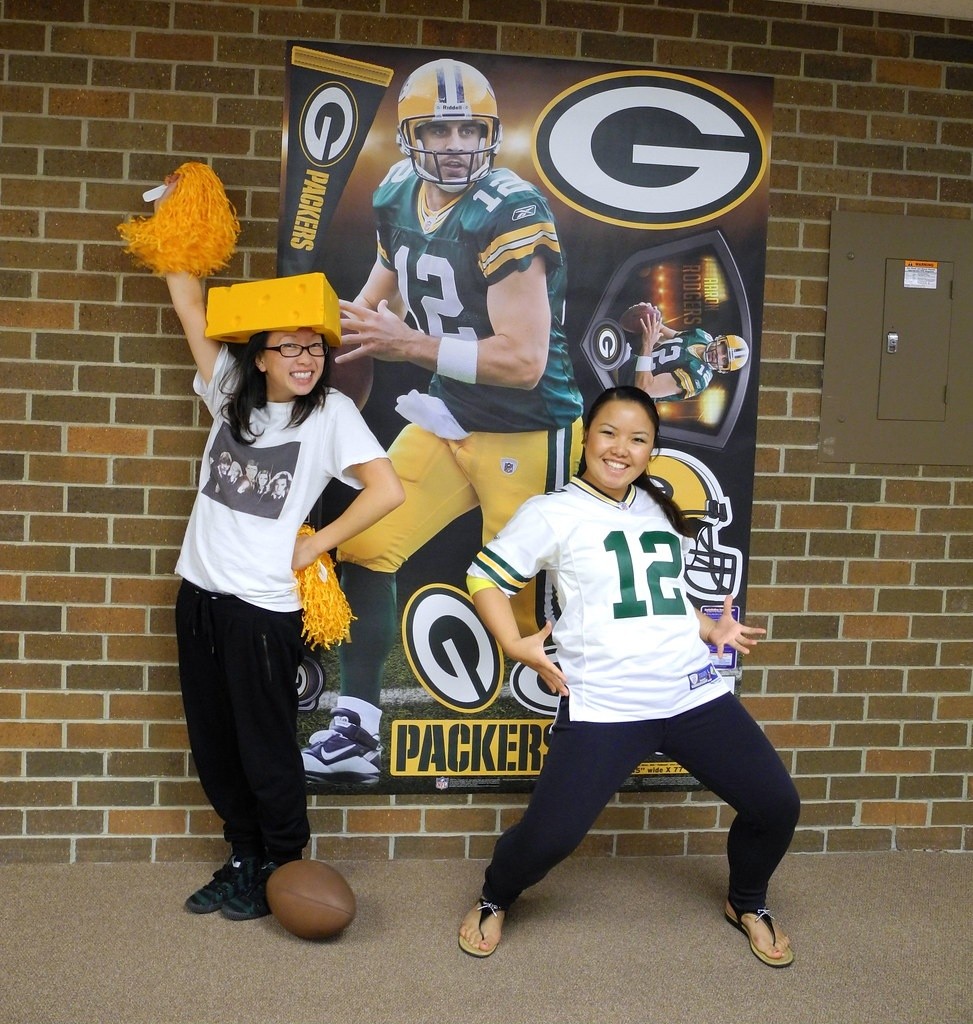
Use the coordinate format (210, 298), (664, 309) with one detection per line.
(433, 335), (481, 386)
(635, 354), (654, 373)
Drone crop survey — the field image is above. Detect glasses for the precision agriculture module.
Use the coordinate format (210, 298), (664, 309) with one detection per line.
(266, 341), (330, 357)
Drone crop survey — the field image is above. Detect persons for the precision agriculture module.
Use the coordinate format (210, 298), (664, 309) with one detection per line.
(632, 301), (749, 405)
(455, 385), (801, 971)
(292, 56), (588, 791)
(141, 167), (410, 922)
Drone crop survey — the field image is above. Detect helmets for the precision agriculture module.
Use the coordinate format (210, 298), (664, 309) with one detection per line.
(399, 57), (498, 168)
(711, 335), (750, 371)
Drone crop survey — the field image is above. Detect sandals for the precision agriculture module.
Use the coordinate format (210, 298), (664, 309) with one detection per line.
(459, 894), (507, 959)
(723, 898), (794, 968)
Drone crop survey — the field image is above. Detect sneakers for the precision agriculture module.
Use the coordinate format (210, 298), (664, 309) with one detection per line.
(186, 853), (280, 922)
(300, 707), (385, 789)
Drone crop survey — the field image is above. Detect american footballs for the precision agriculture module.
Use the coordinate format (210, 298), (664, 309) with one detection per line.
(324, 311), (375, 413)
(264, 859), (356, 941)
